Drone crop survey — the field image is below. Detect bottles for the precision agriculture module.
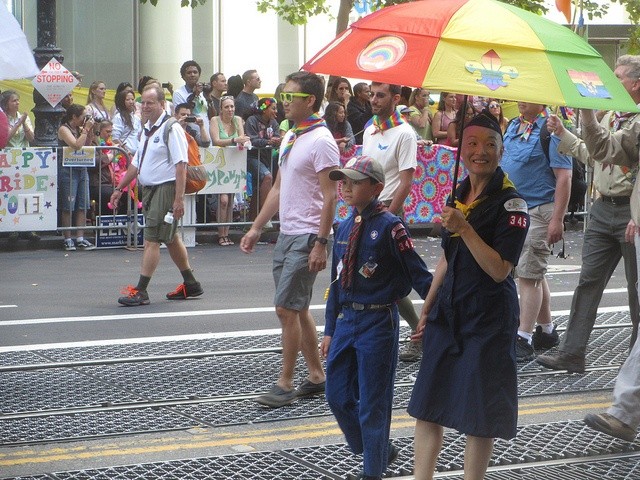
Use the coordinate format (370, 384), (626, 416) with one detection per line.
(164, 209), (174, 224)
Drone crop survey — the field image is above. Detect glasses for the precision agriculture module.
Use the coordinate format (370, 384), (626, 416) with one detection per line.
(219, 95), (234, 101)
(279, 92), (311, 103)
(488, 104), (500, 108)
(339, 87), (349, 90)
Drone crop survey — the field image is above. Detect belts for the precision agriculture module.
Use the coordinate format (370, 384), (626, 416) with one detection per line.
(601, 195), (630, 204)
(342, 301), (396, 311)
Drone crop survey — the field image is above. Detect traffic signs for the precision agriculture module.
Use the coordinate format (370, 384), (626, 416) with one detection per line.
(30, 57), (81, 109)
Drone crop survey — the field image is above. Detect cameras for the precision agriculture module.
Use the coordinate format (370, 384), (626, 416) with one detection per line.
(200, 83), (212, 92)
(83, 115), (101, 123)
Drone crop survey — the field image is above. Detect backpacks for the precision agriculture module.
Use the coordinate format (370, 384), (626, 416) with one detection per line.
(163, 117), (207, 194)
(505, 118), (588, 201)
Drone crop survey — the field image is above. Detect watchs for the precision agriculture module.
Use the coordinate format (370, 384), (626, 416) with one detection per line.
(315, 237), (329, 245)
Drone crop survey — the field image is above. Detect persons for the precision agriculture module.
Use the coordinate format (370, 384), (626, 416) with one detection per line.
(245, 97), (281, 229)
(407, 89), (434, 143)
(272, 83), (285, 121)
(576, 107), (639, 444)
(482, 100), (510, 134)
(110, 82), (136, 114)
(319, 76), (328, 117)
(360, 81), (421, 363)
(0, 89), (35, 148)
(456, 93), (473, 106)
(209, 95), (250, 248)
(83, 80), (111, 121)
(323, 101), (355, 153)
(410, 111), (531, 479)
(321, 158), (433, 477)
(498, 102), (574, 363)
(236, 70), (262, 116)
(537, 54), (640, 374)
(206, 72), (228, 117)
(432, 92), (459, 143)
(400, 87), (412, 107)
(239, 73), (336, 406)
(89, 118), (128, 223)
(136, 76), (174, 102)
(0, 109), (8, 150)
(57, 103), (96, 251)
(173, 103), (210, 148)
(326, 73), (341, 97)
(444, 104), (476, 146)
(330, 78), (352, 103)
(224, 75), (243, 95)
(61, 92), (73, 106)
(110, 88), (142, 156)
(279, 119), (292, 139)
(172, 60), (212, 145)
(109, 84), (204, 306)
(348, 82), (371, 143)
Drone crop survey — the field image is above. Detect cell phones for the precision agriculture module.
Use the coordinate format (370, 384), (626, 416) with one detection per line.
(162, 83), (170, 88)
(184, 116), (195, 122)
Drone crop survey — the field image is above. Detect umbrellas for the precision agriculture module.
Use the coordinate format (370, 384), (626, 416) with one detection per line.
(303, 1), (637, 235)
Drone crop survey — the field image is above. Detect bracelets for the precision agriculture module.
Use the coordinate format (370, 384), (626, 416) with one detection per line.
(24, 129), (31, 133)
(82, 126), (89, 133)
(232, 138), (235, 145)
(114, 187), (124, 192)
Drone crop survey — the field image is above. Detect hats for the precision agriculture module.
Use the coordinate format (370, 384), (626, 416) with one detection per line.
(396, 104), (414, 113)
(462, 107), (504, 143)
(328, 155), (385, 189)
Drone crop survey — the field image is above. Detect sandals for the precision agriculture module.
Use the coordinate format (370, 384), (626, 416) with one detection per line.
(225, 236), (234, 245)
(217, 235), (229, 246)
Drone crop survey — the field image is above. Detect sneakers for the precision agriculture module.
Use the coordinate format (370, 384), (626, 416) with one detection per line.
(75, 240), (96, 251)
(585, 413), (637, 442)
(117, 285), (150, 306)
(398, 329), (424, 361)
(26, 232), (40, 240)
(166, 281), (204, 299)
(63, 241), (76, 251)
(257, 385), (299, 407)
(296, 378), (326, 398)
(515, 334), (536, 362)
(9, 231), (21, 241)
(532, 325), (560, 347)
(535, 347), (584, 372)
(264, 220), (273, 228)
(357, 444), (398, 478)
